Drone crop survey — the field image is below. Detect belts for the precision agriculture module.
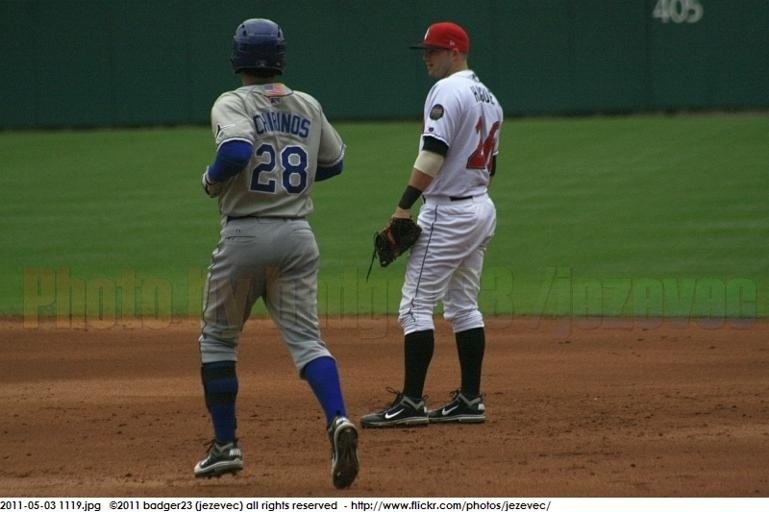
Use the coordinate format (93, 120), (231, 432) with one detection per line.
(422, 194), (473, 204)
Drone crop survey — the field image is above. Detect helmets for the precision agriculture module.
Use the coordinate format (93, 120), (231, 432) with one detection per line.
(231, 17), (286, 75)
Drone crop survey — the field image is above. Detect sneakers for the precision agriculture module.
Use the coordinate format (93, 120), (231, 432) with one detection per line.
(193, 440), (244, 480)
(428, 390), (486, 423)
(327, 414), (360, 489)
(360, 393), (429, 428)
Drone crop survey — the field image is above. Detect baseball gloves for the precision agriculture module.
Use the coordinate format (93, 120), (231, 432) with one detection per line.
(374, 217), (422, 269)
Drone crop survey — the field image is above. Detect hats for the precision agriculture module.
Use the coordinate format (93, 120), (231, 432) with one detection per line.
(409, 22), (470, 55)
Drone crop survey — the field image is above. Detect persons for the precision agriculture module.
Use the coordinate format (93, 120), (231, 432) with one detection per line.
(194, 19), (360, 488)
(358, 23), (503, 427)
